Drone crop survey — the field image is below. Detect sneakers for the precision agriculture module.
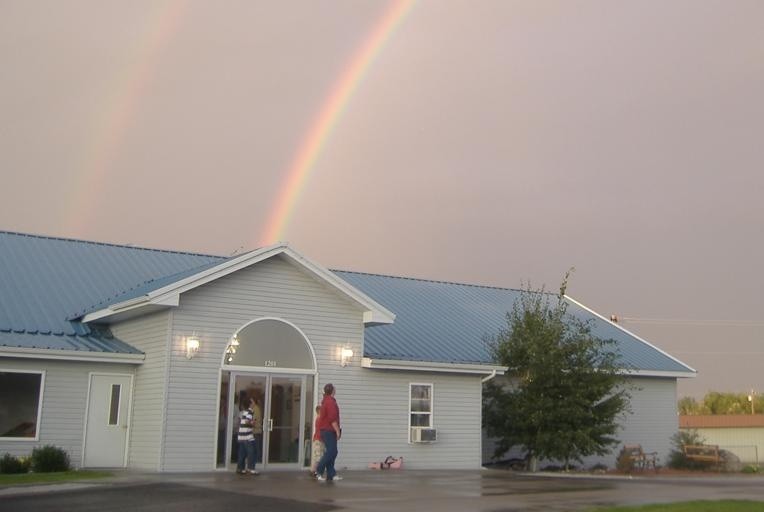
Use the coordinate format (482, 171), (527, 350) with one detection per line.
(236, 469), (260, 475)
(312, 472), (343, 480)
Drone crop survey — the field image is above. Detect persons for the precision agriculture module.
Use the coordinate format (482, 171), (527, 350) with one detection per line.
(219, 391), (263, 475)
(311, 384), (344, 481)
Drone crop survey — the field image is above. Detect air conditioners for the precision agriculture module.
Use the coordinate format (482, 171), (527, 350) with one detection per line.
(411, 427), (437, 443)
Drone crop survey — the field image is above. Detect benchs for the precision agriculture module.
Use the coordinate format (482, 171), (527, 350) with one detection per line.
(682, 444), (726, 463)
(622, 443), (658, 471)
